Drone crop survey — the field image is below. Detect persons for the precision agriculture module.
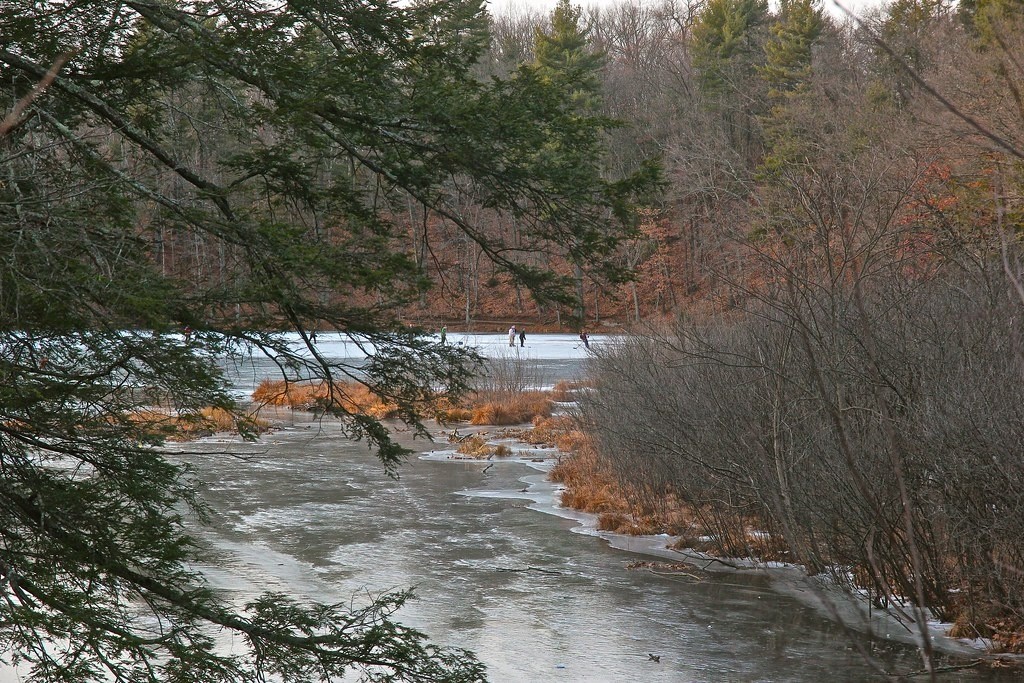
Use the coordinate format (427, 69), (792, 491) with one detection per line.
(579, 331), (589, 348)
(309, 326), (317, 344)
(509, 324), (516, 347)
(184, 324), (191, 340)
(441, 323), (447, 343)
(519, 327), (526, 347)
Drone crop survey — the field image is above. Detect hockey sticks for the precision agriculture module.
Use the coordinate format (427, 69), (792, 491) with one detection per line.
(573, 337), (588, 349)
